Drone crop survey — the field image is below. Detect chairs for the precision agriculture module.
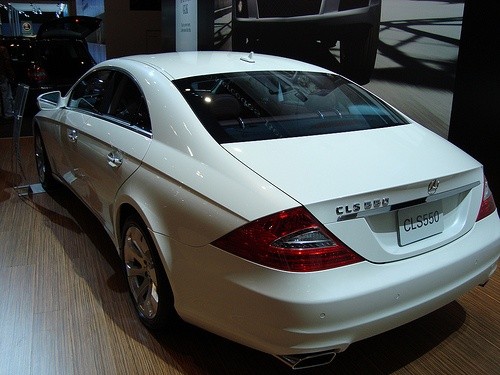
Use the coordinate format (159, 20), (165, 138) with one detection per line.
(199, 72), (343, 122)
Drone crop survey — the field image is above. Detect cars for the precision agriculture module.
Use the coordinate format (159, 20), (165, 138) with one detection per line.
(31, 51), (500, 370)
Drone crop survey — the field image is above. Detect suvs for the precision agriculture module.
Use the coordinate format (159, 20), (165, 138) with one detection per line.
(232, 0), (383, 85)
(0, 16), (102, 138)
